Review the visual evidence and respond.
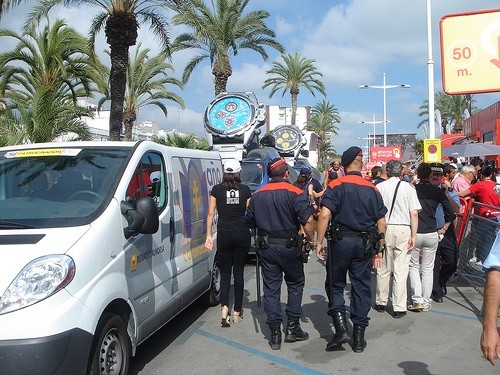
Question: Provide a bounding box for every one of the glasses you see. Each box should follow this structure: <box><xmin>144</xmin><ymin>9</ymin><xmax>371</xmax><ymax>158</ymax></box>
<box><xmin>470</xmin><ymin>171</ymin><xmax>476</xmax><ymax>179</ymax></box>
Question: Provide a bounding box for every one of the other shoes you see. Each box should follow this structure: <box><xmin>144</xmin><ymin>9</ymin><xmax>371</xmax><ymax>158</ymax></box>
<box><xmin>432</xmin><ymin>286</ymin><xmax>447</xmax><ymax>302</ymax></box>
<box><xmin>375</xmin><ymin>304</ymin><xmax>385</xmax><ymax>312</ymax></box>
<box><xmin>394</xmin><ymin>311</ymin><xmax>406</xmax><ymax>318</ymax></box>
<box><xmin>408</xmin><ymin>303</ymin><xmax>431</xmax><ymax>312</ymax></box>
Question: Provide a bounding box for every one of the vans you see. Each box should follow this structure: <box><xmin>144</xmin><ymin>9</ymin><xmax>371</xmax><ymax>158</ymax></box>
<box><xmin>0</xmin><ymin>139</ymin><xmax>226</xmax><ymax>375</ymax></box>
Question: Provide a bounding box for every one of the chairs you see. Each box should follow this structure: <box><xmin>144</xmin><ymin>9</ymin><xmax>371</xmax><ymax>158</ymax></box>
<box><xmin>22</xmin><ymin>172</ymin><xmax>51</xmax><ymax>204</ymax></box>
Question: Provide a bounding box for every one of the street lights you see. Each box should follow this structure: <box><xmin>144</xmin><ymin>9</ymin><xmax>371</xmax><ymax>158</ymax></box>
<box><xmin>357</xmin><ymin>72</ymin><xmax>412</xmax><ymax>148</ymax></box>
<box><xmin>357</xmin><ymin>132</ymin><xmax>382</xmax><ymax>163</ymax></box>
<box><xmin>357</xmin><ymin>113</ymin><xmax>391</xmax><ymax>147</ymax></box>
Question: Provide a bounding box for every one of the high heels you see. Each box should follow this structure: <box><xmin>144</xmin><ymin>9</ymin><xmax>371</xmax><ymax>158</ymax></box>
<box><xmin>233</xmin><ymin>310</ymin><xmax>243</xmax><ymax>323</ymax></box>
<box><xmin>221</xmin><ymin>304</ymin><xmax>231</xmax><ymax>327</ymax></box>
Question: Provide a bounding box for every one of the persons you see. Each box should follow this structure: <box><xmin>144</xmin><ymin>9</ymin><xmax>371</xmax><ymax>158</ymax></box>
<box><xmin>315</xmin><ymin>146</ymin><xmax>388</xmax><ymax>353</ymax></box>
<box><xmin>407</xmin><ymin>163</ymin><xmax>455</xmax><ymax>312</ymax></box>
<box><xmin>245</xmin><ymin>157</ymin><xmax>315</xmax><ymax>349</ymax></box>
<box><xmin>426</xmin><ymin>162</ymin><xmax>461</xmax><ymax>303</ymax></box>
<box><xmin>205</xmin><ymin>159</ymin><xmax>252</xmax><ymax>327</ymax></box>
<box><xmin>373</xmin><ymin>160</ymin><xmax>422</xmax><ymax>318</ymax></box>
<box><xmin>480</xmin><ymin>231</ymin><xmax>500</xmax><ymax>366</ymax></box>
<box><xmin>452</xmin><ymin>157</ymin><xmax>500</xmax><ymax>272</ymax></box>
<box><xmin>289</xmin><ymin>161</ymin><xmax>342</xmax><ymax>248</ymax></box>
<box><xmin>365</xmin><ymin>160</ymin><xmax>455</xmax><ymax>186</ymax></box>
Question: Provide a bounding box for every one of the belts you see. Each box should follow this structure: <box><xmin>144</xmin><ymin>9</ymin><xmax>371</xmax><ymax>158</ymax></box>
<box><xmin>342</xmin><ymin>230</ymin><xmax>362</xmax><ymax>238</ymax></box>
<box><xmin>267</xmin><ymin>237</ymin><xmax>288</xmax><ymax>245</ymax></box>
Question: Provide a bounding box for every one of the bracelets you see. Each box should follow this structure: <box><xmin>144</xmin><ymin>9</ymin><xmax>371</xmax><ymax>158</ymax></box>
<box><xmin>441</xmin><ymin>225</ymin><xmax>448</xmax><ymax>232</ymax></box>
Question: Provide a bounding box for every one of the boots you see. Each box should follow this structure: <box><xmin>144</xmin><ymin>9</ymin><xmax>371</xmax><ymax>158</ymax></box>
<box><xmin>353</xmin><ymin>324</ymin><xmax>367</xmax><ymax>353</ymax></box>
<box><xmin>326</xmin><ymin>311</ymin><xmax>351</xmax><ymax>349</ymax></box>
<box><xmin>285</xmin><ymin>316</ymin><xmax>309</xmax><ymax>340</ymax></box>
<box><xmin>269</xmin><ymin>322</ymin><xmax>282</xmax><ymax>350</ymax></box>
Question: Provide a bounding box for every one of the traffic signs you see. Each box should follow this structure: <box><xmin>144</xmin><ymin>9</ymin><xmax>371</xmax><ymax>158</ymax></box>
<box><xmin>439</xmin><ymin>7</ymin><xmax>500</xmax><ymax>96</ymax></box>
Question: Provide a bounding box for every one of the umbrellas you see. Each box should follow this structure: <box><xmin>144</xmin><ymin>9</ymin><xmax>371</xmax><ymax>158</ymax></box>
<box><xmin>442</xmin><ymin>139</ymin><xmax>500</xmax><ymax>159</ymax></box>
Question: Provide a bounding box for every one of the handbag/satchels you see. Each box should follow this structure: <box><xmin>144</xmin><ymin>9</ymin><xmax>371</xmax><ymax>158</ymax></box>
<box><xmin>365</xmin><ymin>232</ymin><xmax>385</xmax><ymax>256</ymax></box>
<box><xmin>297</xmin><ymin>237</ymin><xmax>310</xmax><ymax>263</ymax></box>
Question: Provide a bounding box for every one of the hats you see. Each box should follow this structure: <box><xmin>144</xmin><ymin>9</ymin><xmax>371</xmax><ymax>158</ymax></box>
<box><xmin>266</xmin><ymin>157</ymin><xmax>285</xmax><ymax>176</ymax></box>
<box><xmin>223</xmin><ymin>159</ymin><xmax>243</xmax><ymax>174</ymax></box>
<box><xmin>300</xmin><ymin>166</ymin><xmax>311</xmax><ymax>175</ymax></box>
<box><xmin>341</xmin><ymin>146</ymin><xmax>363</xmax><ymax>167</ymax></box>
<box><xmin>329</xmin><ymin>172</ymin><xmax>338</xmax><ymax>179</ymax></box>
<box><xmin>430</xmin><ymin>163</ymin><xmax>444</xmax><ymax>174</ymax></box>
